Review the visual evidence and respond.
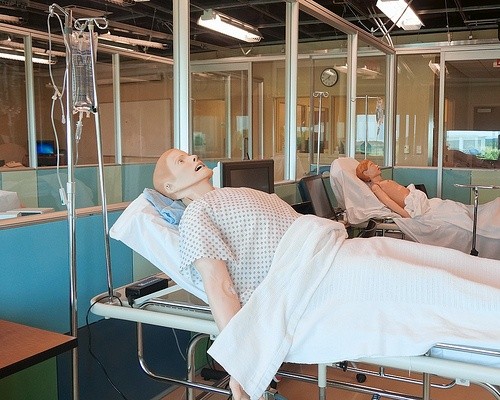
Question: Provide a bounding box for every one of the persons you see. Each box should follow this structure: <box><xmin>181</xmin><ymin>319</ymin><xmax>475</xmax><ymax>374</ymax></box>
<box><xmin>356</xmin><ymin>159</ymin><xmax>411</xmax><ymax>218</ymax></box>
<box><xmin>152</xmin><ymin>148</ymin><xmax>305</xmax><ymax>400</ymax></box>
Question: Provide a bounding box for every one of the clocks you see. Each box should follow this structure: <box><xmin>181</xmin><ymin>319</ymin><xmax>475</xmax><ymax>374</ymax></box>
<box><xmin>320</xmin><ymin>68</ymin><xmax>338</xmax><ymax>87</ymax></box>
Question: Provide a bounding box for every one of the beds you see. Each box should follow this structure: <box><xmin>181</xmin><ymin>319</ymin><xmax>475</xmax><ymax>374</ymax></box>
<box><xmin>88</xmin><ymin>188</ymin><xmax>500</xmax><ymax>400</ymax></box>
<box><xmin>329</xmin><ymin>156</ymin><xmax>500</xmax><ymax>261</ymax></box>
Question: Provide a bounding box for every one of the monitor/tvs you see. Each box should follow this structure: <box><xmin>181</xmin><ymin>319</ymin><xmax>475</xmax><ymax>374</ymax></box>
<box><xmin>36</xmin><ymin>140</ymin><xmax>55</xmax><ymax>155</ymax></box>
<box><xmin>213</xmin><ymin>159</ymin><xmax>275</xmax><ymax>196</ymax></box>
<box><xmin>299</xmin><ymin>174</ymin><xmax>338</xmax><ymax>223</ymax></box>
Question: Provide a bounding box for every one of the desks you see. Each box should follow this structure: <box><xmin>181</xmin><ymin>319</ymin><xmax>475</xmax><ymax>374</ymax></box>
<box><xmin>23</xmin><ymin>155</ymin><xmax>64</xmax><ymax>166</ymax></box>
<box><xmin>0</xmin><ymin>319</ymin><xmax>77</xmax><ymax>379</ymax></box>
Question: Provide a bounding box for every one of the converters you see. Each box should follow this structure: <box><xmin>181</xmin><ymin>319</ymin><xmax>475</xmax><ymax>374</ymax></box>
<box><xmin>125</xmin><ymin>277</ymin><xmax>168</xmax><ymax>299</ymax></box>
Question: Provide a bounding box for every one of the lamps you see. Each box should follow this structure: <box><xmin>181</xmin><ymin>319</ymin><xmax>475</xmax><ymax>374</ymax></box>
<box><xmin>374</xmin><ymin>0</ymin><xmax>425</xmax><ymax>31</ymax></box>
<box><xmin>195</xmin><ymin>8</ymin><xmax>264</xmax><ymax>44</ymax></box>
